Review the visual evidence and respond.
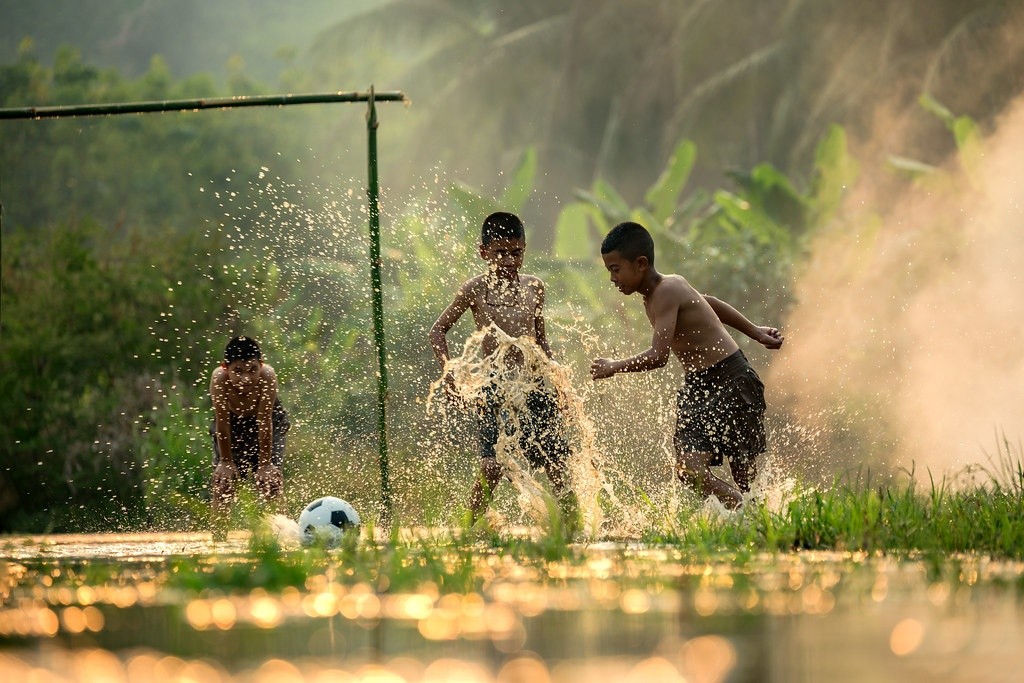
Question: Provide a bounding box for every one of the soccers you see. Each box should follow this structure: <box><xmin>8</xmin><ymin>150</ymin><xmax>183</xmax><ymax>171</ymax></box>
<box><xmin>298</xmin><ymin>497</ymin><xmax>361</xmax><ymax>550</ymax></box>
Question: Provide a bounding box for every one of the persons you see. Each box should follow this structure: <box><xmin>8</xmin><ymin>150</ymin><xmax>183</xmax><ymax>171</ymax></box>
<box><xmin>429</xmin><ymin>212</ymin><xmax>583</xmax><ymax>524</ymax></box>
<box><xmin>210</xmin><ymin>336</ymin><xmax>291</xmax><ymax>541</ymax></box>
<box><xmin>590</xmin><ymin>221</ymin><xmax>784</xmax><ymax>511</ymax></box>
